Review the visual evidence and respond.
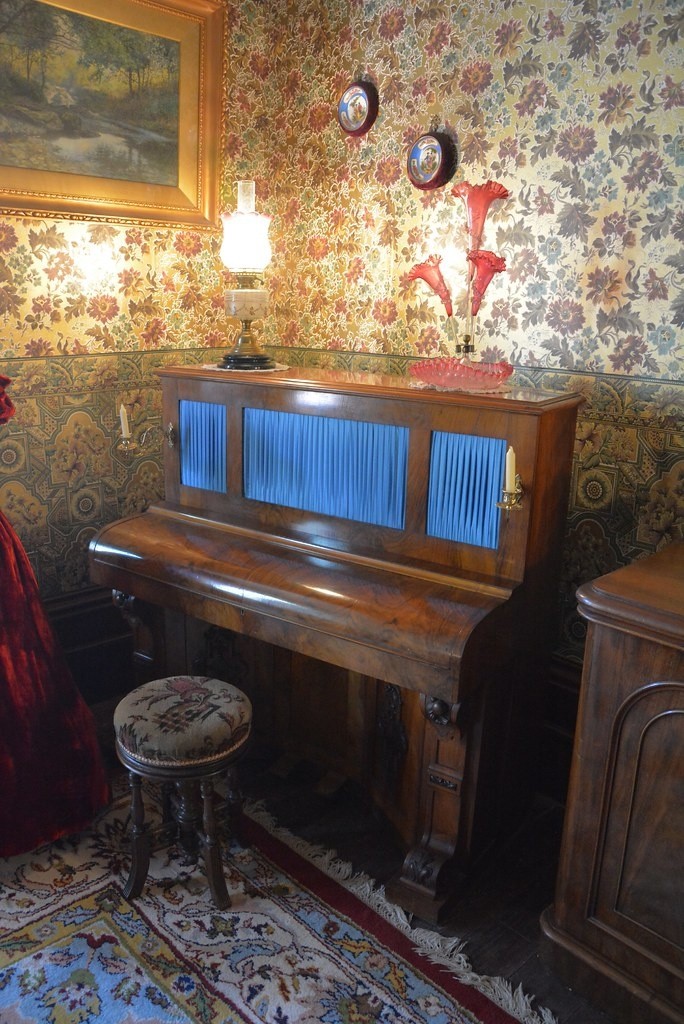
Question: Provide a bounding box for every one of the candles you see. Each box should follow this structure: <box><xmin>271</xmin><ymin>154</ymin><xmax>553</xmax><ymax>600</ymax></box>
<box><xmin>505</xmin><ymin>446</ymin><xmax>516</xmax><ymax>493</ymax></box>
<box><xmin>120</xmin><ymin>403</ymin><xmax>130</xmax><ymax>437</ymax></box>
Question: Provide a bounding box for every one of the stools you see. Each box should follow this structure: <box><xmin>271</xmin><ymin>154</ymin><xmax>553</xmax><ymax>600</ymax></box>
<box><xmin>112</xmin><ymin>676</ymin><xmax>254</xmax><ymax>911</ymax></box>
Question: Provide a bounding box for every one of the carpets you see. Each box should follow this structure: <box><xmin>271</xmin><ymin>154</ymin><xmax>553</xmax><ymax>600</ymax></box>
<box><xmin>0</xmin><ymin>763</ymin><xmax>563</xmax><ymax>1024</ymax></box>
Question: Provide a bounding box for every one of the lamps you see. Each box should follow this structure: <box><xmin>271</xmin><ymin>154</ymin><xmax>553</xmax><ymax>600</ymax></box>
<box><xmin>221</xmin><ymin>180</ymin><xmax>277</xmax><ymax>370</ymax></box>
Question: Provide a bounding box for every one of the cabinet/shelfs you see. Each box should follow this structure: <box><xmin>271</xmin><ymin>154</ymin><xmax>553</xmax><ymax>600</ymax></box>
<box><xmin>540</xmin><ymin>543</ymin><xmax>684</xmax><ymax>1024</ymax></box>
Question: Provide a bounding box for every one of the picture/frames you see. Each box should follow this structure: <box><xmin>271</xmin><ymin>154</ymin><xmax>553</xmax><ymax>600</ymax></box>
<box><xmin>2</xmin><ymin>1</ymin><xmax>231</xmax><ymax>229</ymax></box>
